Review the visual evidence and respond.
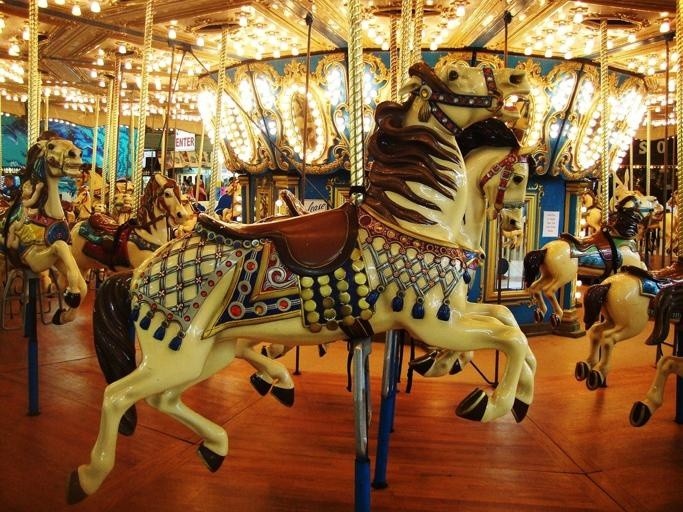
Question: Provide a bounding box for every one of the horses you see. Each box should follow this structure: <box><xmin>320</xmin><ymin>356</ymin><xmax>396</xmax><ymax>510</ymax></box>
<box><xmin>522</xmin><ymin>193</ymin><xmax>659</xmax><ymax>327</ymax></box>
<box><xmin>231</xmin><ymin>119</ymin><xmax>531</xmax><ymax>408</ymax></box>
<box><xmin>629</xmin><ymin>278</ymin><xmax>683</xmax><ymax>427</ymax></box>
<box><xmin>574</xmin><ymin>256</ymin><xmax>683</xmax><ymax>395</ymax></box>
<box><xmin>173</xmin><ymin>193</ymin><xmax>205</xmax><ymax>237</ymax></box>
<box><xmin>0</xmin><ymin>130</ymin><xmax>87</xmax><ymax>326</ymax></box>
<box><xmin>87</xmin><ymin>171</ymin><xmax>134</xmax><ymax>206</ymax></box>
<box><xmin>55</xmin><ymin>169</ymin><xmax>189</xmax><ymax>290</ymax></box>
<box><xmin>72</xmin><ymin>187</ymin><xmax>93</xmax><ymax>219</ymax></box>
<box><xmin>61</xmin><ymin>51</ymin><xmax>535</xmax><ymax>509</ymax></box>
<box><xmin>222</xmin><ymin>176</ymin><xmax>243</xmax><ymax>223</ymax></box>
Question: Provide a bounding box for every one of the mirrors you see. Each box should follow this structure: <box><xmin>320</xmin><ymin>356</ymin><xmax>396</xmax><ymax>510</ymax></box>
<box><xmin>326</xmin><ymin>177</ymin><xmax>350</xmax><ymax>209</ymax></box>
<box><xmin>255</xmin><ymin>188</ymin><xmax>273</xmax><ymax>221</ymax></box>
<box><xmin>477</xmin><ymin>183</ymin><xmax>545</xmax><ymax>308</ymax></box>
